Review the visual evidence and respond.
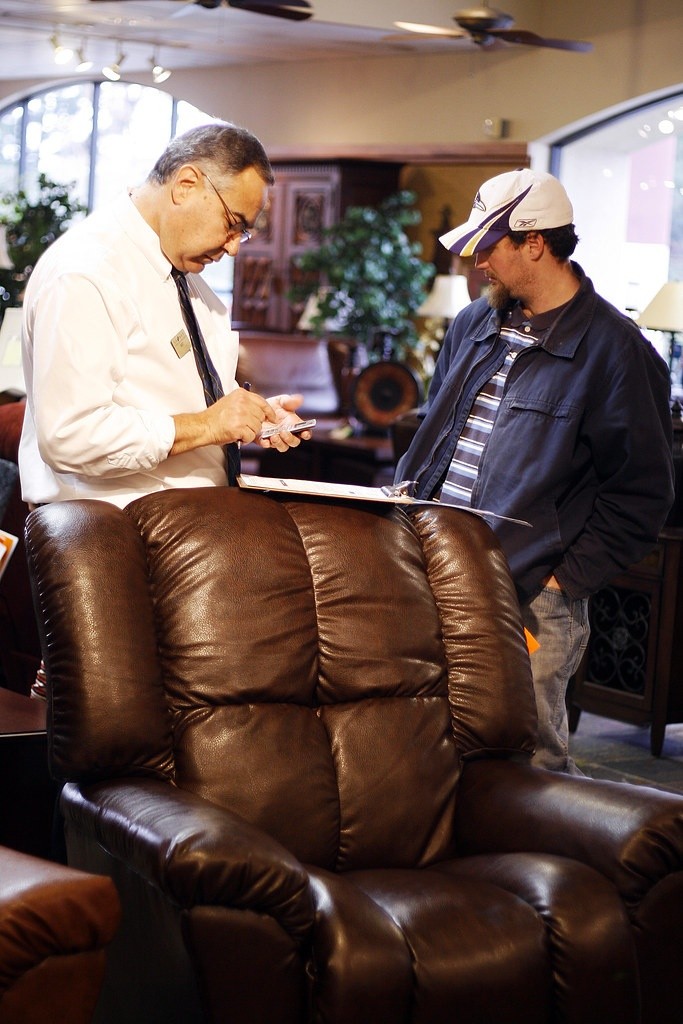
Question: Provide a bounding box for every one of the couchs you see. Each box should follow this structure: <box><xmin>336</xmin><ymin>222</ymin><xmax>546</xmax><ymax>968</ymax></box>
<box><xmin>21</xmin><ymin>488</ymin><xmax>683</xmax><ymax>1024</ymax></box>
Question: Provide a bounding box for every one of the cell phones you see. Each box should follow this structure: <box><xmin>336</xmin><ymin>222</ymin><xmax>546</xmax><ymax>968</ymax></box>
<box><xmin>262</xmin><ymin>418</ymin><xmax>317</xmax><ymax>439</ymax></box>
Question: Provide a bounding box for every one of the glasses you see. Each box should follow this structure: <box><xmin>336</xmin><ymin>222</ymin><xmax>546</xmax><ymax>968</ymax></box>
<box><xmin>199</xmin><ymin>171</ymin><xmax>253</xmax><ymax>246</ymax></box>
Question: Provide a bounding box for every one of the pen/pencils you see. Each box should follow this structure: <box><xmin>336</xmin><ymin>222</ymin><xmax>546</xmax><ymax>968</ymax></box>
<box><xmin>238</xmin><ymin>381</ymin><xmax>250</xmax><ymax>450</ymax></box>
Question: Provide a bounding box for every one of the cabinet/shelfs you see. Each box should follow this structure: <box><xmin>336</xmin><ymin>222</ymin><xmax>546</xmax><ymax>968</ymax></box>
<box><xmin>232</xmin><ymin>153</ymin><xmax>407</xmax><ymax>331</ymax></box>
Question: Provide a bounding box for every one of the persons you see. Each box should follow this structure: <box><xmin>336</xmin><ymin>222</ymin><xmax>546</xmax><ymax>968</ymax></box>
<box><xmin>17</xmin><ymin>114</ymin><xmax>311</xmax><ymax>511</ymax></box>
<box><xmin>394</xmin><ymin>166</ymin><xmax>679</xmax><ymax>769</ymax></box>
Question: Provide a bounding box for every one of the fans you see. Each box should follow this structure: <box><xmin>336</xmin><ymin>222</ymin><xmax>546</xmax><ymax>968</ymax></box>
<box><xmin>93</xmin><ymin>0</ymin><xmax>312</xmax><ymax>23</ymax></box>
<box><xmin>391</xmin><ymin>0</ymin><xmax>591</xmax><ymax>54</ymax></box>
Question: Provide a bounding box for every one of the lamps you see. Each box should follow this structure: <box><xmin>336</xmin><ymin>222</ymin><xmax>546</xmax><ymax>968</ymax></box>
<box><xmin>148</xmin><ymin>45</ymin><xmax>172</xmax><ymax>83</ymax></box>
<box><xmin>48</xmin><ymin>32</ymin><xmax>74</xmax><ymax>68</ymax></box>
<box><xmin>70</xmin><ymin>36</ymin><xmax>93</xmax><ymax>71</ymax></box>
<box><xmin>102</xmin><ymin>41</ymin><xmax>128</xmax><ymax>82</ymax></box>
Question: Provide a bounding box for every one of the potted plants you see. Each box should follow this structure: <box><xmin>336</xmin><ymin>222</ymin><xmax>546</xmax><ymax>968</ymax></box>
<box><xmin>290</xmin><ymin>191</ymin><xmax>436</xmax><ymax>405</ymax></box>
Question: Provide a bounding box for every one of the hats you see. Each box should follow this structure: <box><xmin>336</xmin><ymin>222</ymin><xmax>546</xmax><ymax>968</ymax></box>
<box><xmin>438</xmin><ymin>166</ymin><xmax>574</xmax><ymax>258</ymax></box>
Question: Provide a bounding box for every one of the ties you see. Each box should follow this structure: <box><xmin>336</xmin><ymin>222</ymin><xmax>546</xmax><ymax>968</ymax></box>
<box><xmin>168</xmin><ymin>267</ymin><xmax>242</xmax><ymax>486</ymax></box>
